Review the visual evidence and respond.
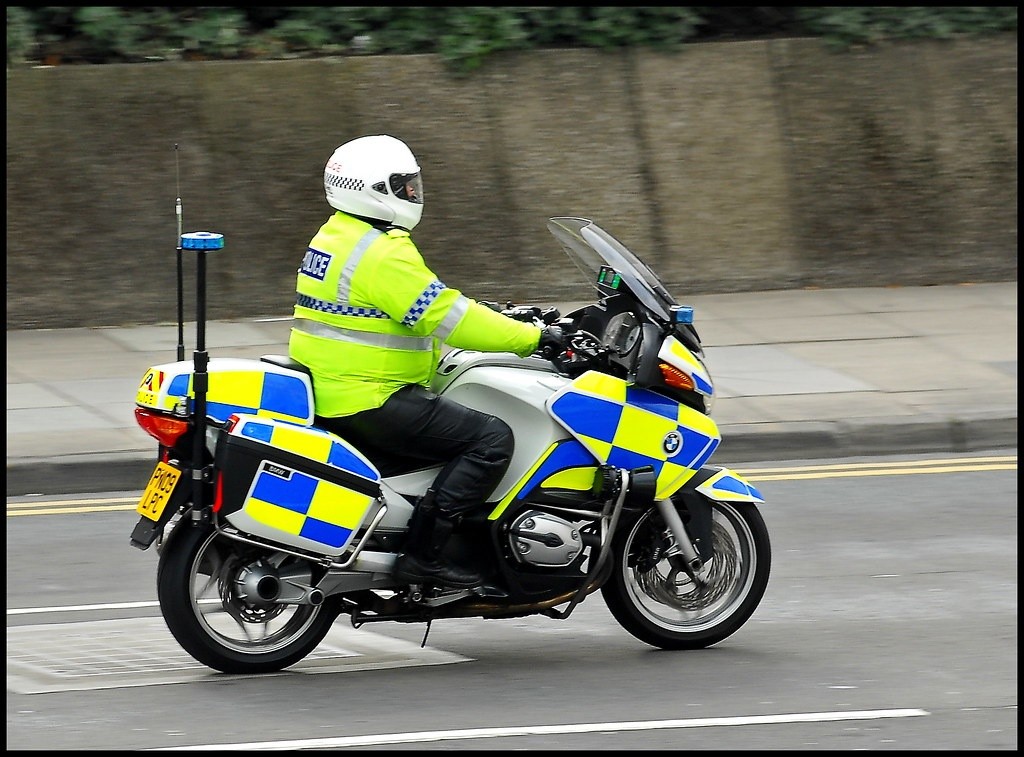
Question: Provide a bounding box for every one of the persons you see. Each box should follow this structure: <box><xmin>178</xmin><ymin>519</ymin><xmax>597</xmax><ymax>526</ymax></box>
<box><xmin>292</xmin><ymin>135</ymin><xmax>570</xmax><ymax>591</ymax></box>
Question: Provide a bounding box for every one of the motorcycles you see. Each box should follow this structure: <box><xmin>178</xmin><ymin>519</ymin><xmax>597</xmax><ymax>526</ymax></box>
<box><xmin>126</xmin><ymin>142</ymin><xmax>774</xmax><ymax>673</ymax></box>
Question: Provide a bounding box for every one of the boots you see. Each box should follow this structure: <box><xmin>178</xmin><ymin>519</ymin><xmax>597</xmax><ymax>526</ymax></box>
<box><xmin>391</xmin><ymin>495</ymin><xmax>484</xmax><ymax>590</ymax></box>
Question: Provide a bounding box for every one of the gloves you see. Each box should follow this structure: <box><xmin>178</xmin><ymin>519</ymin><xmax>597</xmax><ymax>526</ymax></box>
<box><xmin>539</xmin><ymin>323</ymin><xmax>567</xmax><ymax>361</ymax></box>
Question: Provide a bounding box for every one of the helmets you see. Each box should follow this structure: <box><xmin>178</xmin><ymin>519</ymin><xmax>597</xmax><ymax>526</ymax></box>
<box><xmin>323</xmin><ymin>134</ymin><xmax>424</xmax><ymax>230</ymax></box>
